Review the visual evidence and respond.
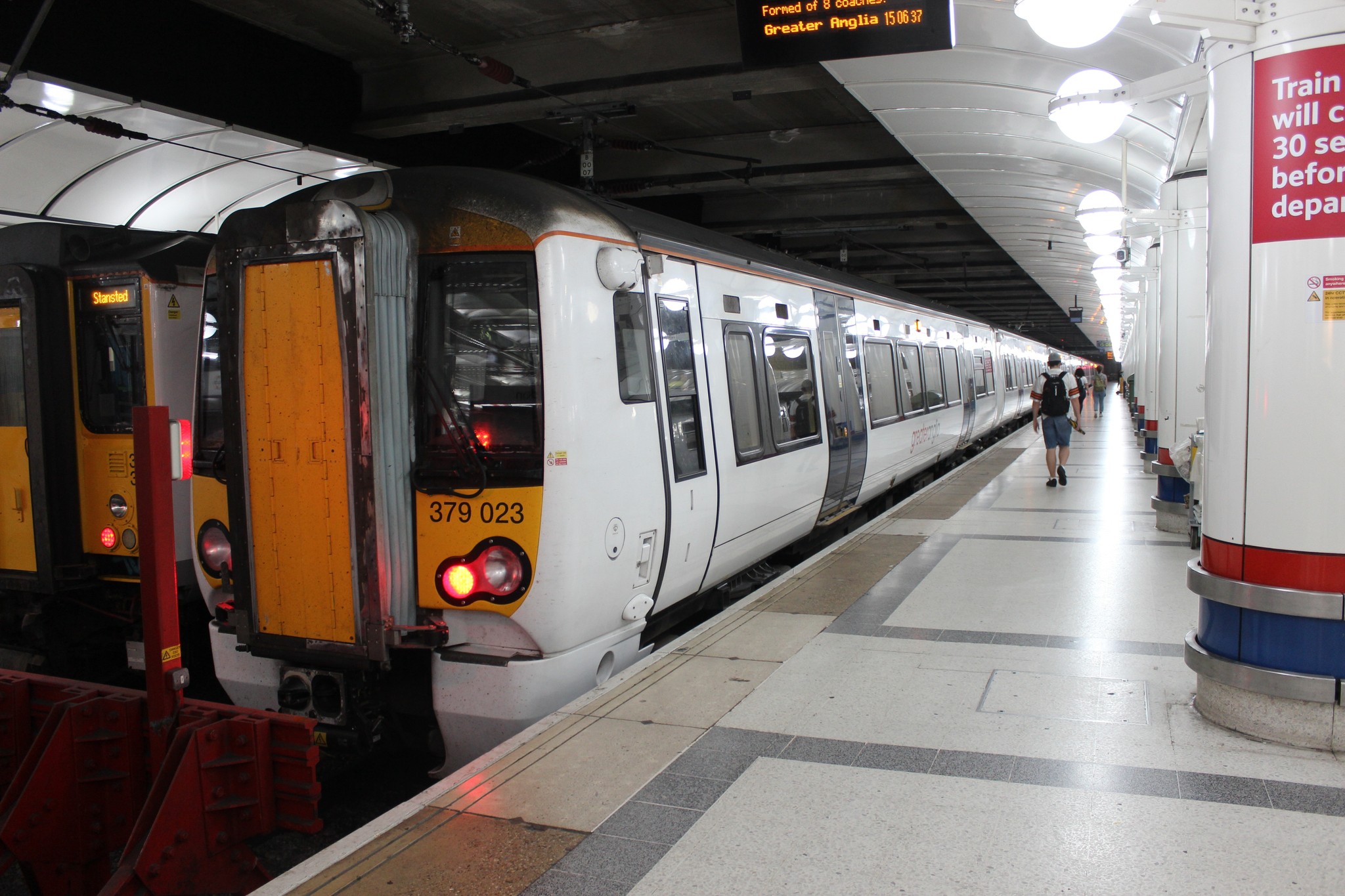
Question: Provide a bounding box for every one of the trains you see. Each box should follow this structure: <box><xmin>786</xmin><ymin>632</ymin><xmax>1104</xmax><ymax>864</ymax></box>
<box><xmin>185</xmin><ymin>161</ymin><xmax>1103</xmax><ymax>780</ymax></box>
<box><xmin>0</xmin><ymin>221</ymin><xmax>231</xmax><ymax>674</ymax></box>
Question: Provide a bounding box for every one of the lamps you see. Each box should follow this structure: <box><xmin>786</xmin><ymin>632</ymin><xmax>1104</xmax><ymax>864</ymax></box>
<box><xmin>1013</xmin><ymin>0</ymin><xmax>1196</xmax><ymax>66</ymax></box>
<box><xmin>1075</xmin><ymin>190</ymin><xmax>1180</xmax><ymax>363</ymax></box>
<box><xmin>1049</xmin><ymin>60</ymin><xmax>1209</xmax><ymax>144</ymax></box>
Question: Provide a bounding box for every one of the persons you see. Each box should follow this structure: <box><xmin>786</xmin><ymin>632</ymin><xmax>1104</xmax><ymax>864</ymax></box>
<box><xmin>1029</xmin><ymin>352</ymin><xmax>1081</xmax><ymax>488</ymax></box>
<box><xmin>1117</xmin><ymin>370</ymin><xmax>1124</xmax><ymax>388</ymax></box>
<box><xmin>1090</xmin><ymin>365</ymin><xmax>1108</xmax><ymax>418</ymax></box>
<box><xmin>1074</xmin><ymin>368</ymin><xmax>1090</xmax><ymax>415</ymax></box>
<box><xmin>788</xmin><ymin>379</ymin><xmax>817</xmax><ymax>441</ymax></box>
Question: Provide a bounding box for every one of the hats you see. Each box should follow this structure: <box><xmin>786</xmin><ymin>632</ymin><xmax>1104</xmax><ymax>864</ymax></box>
<box><xmin>1048</xmin><ymin>352</ymin><xmax>1062</xmax><ymax>366</ymax></box>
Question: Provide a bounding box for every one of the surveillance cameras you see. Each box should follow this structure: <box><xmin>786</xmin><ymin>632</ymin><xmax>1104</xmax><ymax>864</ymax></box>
<box><xmin>1116</xmin><ymin>247</ymin><xmax>1131</xmax><ymax>261</ymax></box>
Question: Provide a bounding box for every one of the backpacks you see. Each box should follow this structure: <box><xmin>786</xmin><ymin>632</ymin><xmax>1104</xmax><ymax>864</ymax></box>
<box><xmin>1040</xmin><ymin>371</ymin><xmax>1070</xmax><ymax>416</ymax></box>
<box><xmin>1075</xmin><ymin>376</ymin><xmax>1084</xmax><ymax>392</ymax></box>
<box><xmin>1094</xmin><ymin>374</ymin><xmax>1105</xmax><ymax>392</ymax></box>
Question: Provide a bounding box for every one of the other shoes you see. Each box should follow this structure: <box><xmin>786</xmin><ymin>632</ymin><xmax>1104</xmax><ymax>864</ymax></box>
<box><xmin>1094</xmin><ymin>411</ymin><xmax>1097</xmax><ymax>418</ymax></box>
<box><xmin>1057</xmin><ymin>465</ymin><xmax>1067</xmax><ymax>486</ymax></box>
<box><xmin>1099</xmin><ymin>413</ymin><xmax>1103</xmax><ymax>417</ymax></box>
<box><xmin>1046</xmin><ymin>478</ymin><xmax>1057</xmax><ymax>487</ymax></box>
